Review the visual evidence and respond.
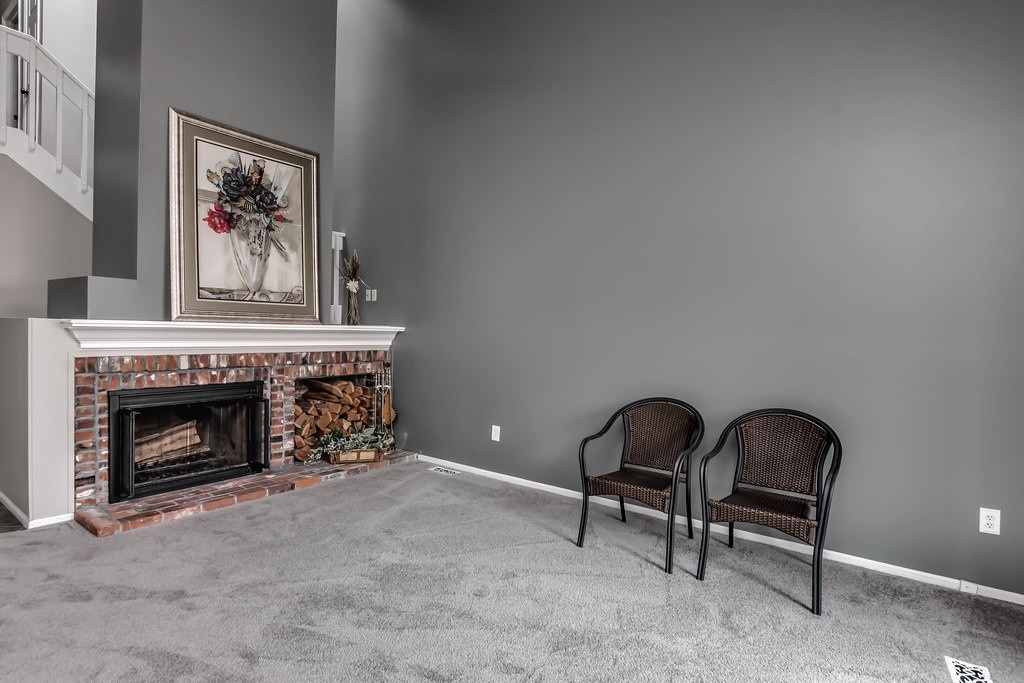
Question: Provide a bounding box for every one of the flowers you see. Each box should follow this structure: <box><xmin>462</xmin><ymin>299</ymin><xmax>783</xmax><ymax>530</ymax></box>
<box><xmin>202</xmin><ymin>151</ymin><xmax>294</xmax><ymax>263</ymax></box>
<box><xmin>338</xmin><ymin>248</ymin><xmax>370</xmax><ymax>294</ymax></box>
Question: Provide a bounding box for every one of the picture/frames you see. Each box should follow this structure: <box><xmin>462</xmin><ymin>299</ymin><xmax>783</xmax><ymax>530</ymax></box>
<box><xmin>168</xmin><ymin>106</ymin><xmax>322</xmax><ymax>326</ymax></box>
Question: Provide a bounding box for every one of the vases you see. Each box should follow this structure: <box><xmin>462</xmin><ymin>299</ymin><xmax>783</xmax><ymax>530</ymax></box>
<box><xmin>347</xmin><ymin>290</ymin><xmax>360</xmax><ymax>324</ymax></box>
<box><xmin>230</xmin><ymin>216</ymin><xmax>276</xmax><ymax>294</ymax></box>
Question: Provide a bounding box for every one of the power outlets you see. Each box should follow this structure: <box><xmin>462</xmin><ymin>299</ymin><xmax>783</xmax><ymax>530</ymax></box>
<box><xmin>491</xmin><ymin>425</ymin><xmax>500</xmax><ymax>441</ymax></box>
<box><xmin>978</xmin><ymin>508</ymin><xmax>1003</xmax><ymax>536</ymax></box>
<box><xmin>365</xmin><ymin>290</ymin><xmax>372</xmax><ymax>302</ymax></box>
<box><xmin>372</xmin><ymin>289</ymin><xmax>377</xmax><ymax>301</ymax></box>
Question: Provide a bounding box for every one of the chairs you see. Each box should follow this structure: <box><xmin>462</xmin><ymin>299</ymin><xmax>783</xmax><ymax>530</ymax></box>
<box><xmin>576</xmin><ymin>396</ymin><xmax>706</xmax><ymax>575</ymax></box>
<box><xmin>696</xmin><ymin>407</ymin><xmax>843</xmax><ymax>616</ymax></box>
